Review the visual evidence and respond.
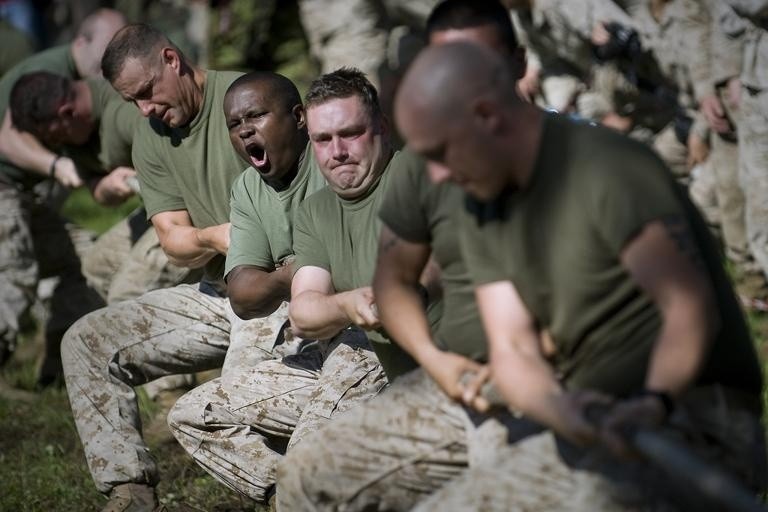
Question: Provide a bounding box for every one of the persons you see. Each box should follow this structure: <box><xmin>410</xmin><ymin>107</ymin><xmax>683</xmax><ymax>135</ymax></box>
<box><xmin>275</xmin><ymin>2</ymin><xmax>527</xmax><ymax>512</ymax></box>
<box><xmin>0</xmin><ymin>2</ymin><xmax>425</xmax><ymax>511</ymax></box>
<box><xmin>394</xmin><ymin>40</ymin><xmax>767</xmax><ymax>510</ymax></box>
<box><xmin>511</xmin><ymin>1</ymin><xmax>767</xmax><ymax>309</ymax></box>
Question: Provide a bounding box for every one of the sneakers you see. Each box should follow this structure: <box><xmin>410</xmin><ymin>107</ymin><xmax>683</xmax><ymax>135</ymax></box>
<box><xmin>102</xmin><ymin>483</ymin><xmax>168</xmax><ymax>512</ymax></box>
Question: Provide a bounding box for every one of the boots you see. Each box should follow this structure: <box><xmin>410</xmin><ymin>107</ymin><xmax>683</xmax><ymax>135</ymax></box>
<box><xmin>142</xmin><ymin>387</ymin><xmax>191</xmax><ymax>447</ymax></box>
